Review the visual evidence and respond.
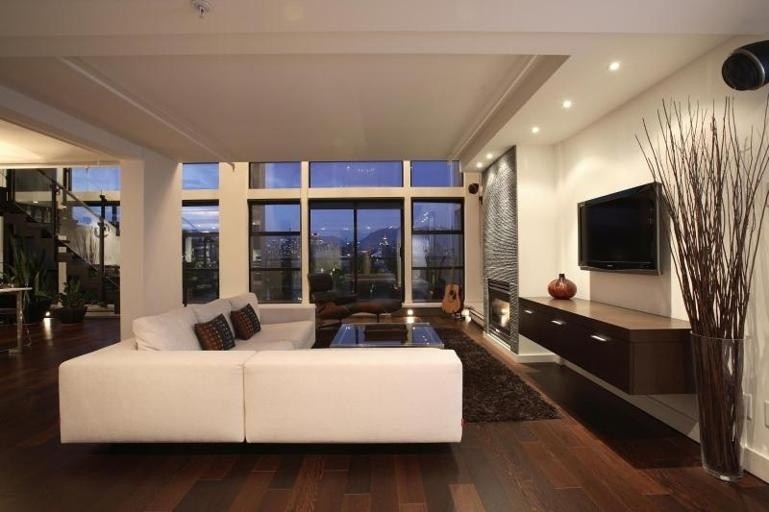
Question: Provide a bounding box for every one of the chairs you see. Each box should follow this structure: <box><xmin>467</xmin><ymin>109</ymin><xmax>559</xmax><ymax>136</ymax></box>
<box><xmin>308</xmin><ymin>273</ymin><xmax>402</xmax><ymax>323</ymax></box>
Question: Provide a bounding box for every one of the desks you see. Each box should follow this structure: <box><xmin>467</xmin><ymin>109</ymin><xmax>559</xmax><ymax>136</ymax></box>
<box><xmin>0</xmin><ymin>286</ymin><xmax>33</xmax><ymax>324</ymax></box>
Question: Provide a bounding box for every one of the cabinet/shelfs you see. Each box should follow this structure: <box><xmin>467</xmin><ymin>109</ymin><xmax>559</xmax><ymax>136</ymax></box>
<box><xmin>518</xmin><ymin>296</ymin><xmax>691</xmax><ymax>395</ymax></box>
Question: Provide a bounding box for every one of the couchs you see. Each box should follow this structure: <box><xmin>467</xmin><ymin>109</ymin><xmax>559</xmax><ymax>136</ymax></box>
<box><xmin>58</xmin><ymin>303</ymin><xmax>463</xmax><ymax>444</ymax></box>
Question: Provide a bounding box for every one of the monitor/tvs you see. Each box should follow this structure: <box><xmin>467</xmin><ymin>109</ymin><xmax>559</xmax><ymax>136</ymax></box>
<box><xmin>577</xmin><ymin>182</ymin><xmax>664</xmax><ymax>277</ymax></box>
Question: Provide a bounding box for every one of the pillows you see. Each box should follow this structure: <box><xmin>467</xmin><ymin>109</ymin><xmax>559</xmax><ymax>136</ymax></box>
<box><xmin>229</xmin><ymin>293</ymin><xmax>260</xmax><ymax>322</ymax></box>
<box><xmin>230</xmin><ymin>304</ymin><xmax>260</xmax><ymax>340</ymax></box>
<box><xmin>189</xmin><ymin>298</ymin><xmax>235</xmax><ymax>338</ymax></box>
<box><xmin>194</xmin><ymin>313</ymin><xmax>235</xmax><ymax>350</ymax></box>
<box><xmin>132</xmin><ymin>307</ymin><xmax>201</xmax><ymax>351</ymax></box>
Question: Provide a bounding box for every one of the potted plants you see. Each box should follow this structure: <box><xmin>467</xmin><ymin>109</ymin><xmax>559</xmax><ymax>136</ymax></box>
<box><xmin>630</xmin><ymin>95</ymin><xmax>769</xmax><ymax>481</ymax></box>
<box><xmin>60</xmin><ymin>275</ymin><xmax>88</xmax><ymax>324</ymax></box>
<box><xmin>6</xmin><ymin>248</ymin><xmax>53</xmax><ymax>323</ymax></box>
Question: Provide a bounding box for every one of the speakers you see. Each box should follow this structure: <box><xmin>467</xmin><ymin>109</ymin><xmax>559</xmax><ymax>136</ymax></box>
<box><xmin>468</xmin><ymin>183</ymin><xmax>479</xmax><ymax>194</ymax></box>
<box><xmin>722</xmin><ymin>40</ymin><xmax>769</xmax><ymax>91</ymax></box>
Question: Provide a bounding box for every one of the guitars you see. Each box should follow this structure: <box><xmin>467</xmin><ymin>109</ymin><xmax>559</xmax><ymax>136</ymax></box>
<box><xmin>442</xmin><ymin>258</ymin><xmax>460</xmax><ymax>313</ymax></box>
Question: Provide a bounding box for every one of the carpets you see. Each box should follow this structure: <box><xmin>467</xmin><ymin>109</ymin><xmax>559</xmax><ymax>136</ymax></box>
<box><xmin>311</xmin><ymin>328</ymin><xmax>562</xmax><ymax>422</ymax></box>
